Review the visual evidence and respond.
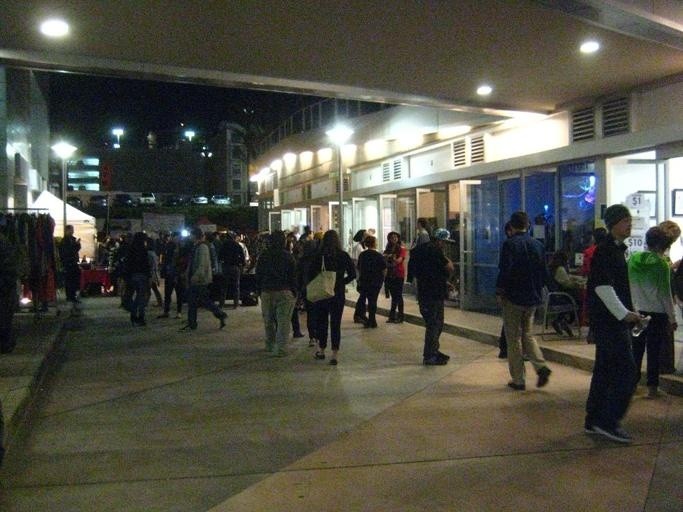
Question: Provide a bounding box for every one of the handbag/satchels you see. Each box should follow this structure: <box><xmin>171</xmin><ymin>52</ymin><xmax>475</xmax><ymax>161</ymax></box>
<box><xmin>304</xmin><ymin>273</ymin><xmax>336</xmax><ymax>301</ymax></box>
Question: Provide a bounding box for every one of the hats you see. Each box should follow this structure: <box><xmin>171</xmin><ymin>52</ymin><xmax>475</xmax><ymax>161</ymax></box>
<box><xmin>432</xmin><ymin>227</ymin><xmax>455</xmax><ymax>243</ymax></box>
<box><xmin>604</xmin><ymin>206</ymin><xmax>628</xmax><ymax>228</ymax></box>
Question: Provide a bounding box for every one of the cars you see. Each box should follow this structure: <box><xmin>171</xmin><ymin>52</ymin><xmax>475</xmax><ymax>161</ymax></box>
<box><xmin>162</xmin><ymin>195</ymin><xmax>231</xmax><ymax>207</ymax></box>
<box><xmin>108</xmin><ymin>194</ymin><xmax>133</xmax><ymax>208</ymax></box>
<box><xmin>88</xmin><ymin>195</ymin><xmax>108</xmax><ymax>208</ymax></box>
<box><xmin>67</xmin><ymin>197</ymin><xmax>82</xmax><ymax>209</ymax></box>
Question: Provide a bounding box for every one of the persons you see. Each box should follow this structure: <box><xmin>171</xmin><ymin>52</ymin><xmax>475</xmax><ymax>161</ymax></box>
<box><xmin>60</xmin><ymin>225</ymin><xmax>82</xmax><ymax>304</ymax></box>
<box><xmin>411</xmin><ymin>228</ymin><xmax>455</xmax><ymax>365</ymax></box>
<box><xmin>351</xmin><ymin>229</ymin><xmax>407</xmax><ymax>328</ymax></box>
<box><xmin>585</xmin><ymin>204</ymin><xmax>644</xmax><ymax>445</ymax></box>
<box><xmin>254</xmin><ymin>229</ymin><xmax>357</xmax><ymax>366</ymax></box>
<box><xmin>499</xmin><ymin>211</ymin><xmax>552</xmax><ymax>390</ymax></box>
<box><xmin>583</xmin><ymin>226</ymin><xmax>608</xmax><ymax>344</ymax></box>
<box><xmin>545</xmin><ymin>247</ymin><xmax>582</xmax><ymax>336</ymax></box>
<box><xmin>415</xmin><ymin>217</ymin><xmax>430</xmax><ymax>247</ymax></box>
<box><xmin>625</xmin><ymin>220</ymin><xmax>681</xmax><ymax>398</ymax></box>
<box><xmin>100</xmin><ymin>227</ymin><xmax>259</xmax><ymax>332</ymax></box>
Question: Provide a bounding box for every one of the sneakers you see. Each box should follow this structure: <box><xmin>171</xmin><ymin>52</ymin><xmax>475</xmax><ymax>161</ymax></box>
<box><xmin>537</xmin><ymin>366</ymin><xmax>550</xmax><ymax>388</ymax></box>
<box><xmin>510</xmin><ymin>381</ymin><xmax>527</xmax><ymax>391</ymax></box>
<box><xmin>423</xmin><ymin>353</ymin><xmax>451</xmax><ymax>366</ymax></box>
<box><xmin>316</xmin><ymin>351</ymin><xmax>325</xmax><ymax>360</ymax></box>
<box><xmin>584</xmin><ymin>424</ymin><xmax>635</xmax><ymax>445</ymax></box>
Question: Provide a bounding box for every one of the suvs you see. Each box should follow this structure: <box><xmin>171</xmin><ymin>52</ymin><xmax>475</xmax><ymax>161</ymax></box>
<box><xmin>136</xmin><ymin>192</ymin><xmax>156</xmax><ymax>207</ymax></box>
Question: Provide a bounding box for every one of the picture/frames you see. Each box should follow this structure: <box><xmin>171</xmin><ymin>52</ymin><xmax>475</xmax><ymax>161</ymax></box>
<box><xmin>672</xmin><ymin>189</ymin><xmax>683</xmax><ymax>218</ymax></box>
<box><xmin>637</xmin><ymin>190</ymin><xmax>658</xmax><ymax>218</ymax></box>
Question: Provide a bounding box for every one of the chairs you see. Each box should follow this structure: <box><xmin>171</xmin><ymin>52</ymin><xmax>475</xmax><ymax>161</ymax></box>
<box><xmin>538</xmin><ymin>285</ymin><xmax>582</xmax><ymax>339</ymax></box>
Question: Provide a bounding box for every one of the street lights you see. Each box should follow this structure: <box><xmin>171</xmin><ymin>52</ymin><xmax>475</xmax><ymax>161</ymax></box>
<box><xmin>326</xmin><ymin>121</ymin><xmax>351</xmax><ymax>248</ymax></box>
<box><xmin>243</xmin><ymin>106</ymin><xmax>254</xmax><ymax>204</ymax></box>
<box><xmin>51</xmin><ymin>140</ymin><xmax>80</xmax><ymax>236</ymax></box>
<box><xmin>199</xmin><ymin>145</ymin><xmax>212</xmax><ymax>202</ymax></box>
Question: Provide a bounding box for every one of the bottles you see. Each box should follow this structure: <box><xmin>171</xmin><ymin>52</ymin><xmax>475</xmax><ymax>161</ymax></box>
<box><xmin>81</xmin><ymin>255</ymin><xmax>87</xmax><ymax>263</ymax></box>
<box><xmin>631</xmin><ymin>315</ymin><xmax>652</xmax><ymax>339</ymax></box>
